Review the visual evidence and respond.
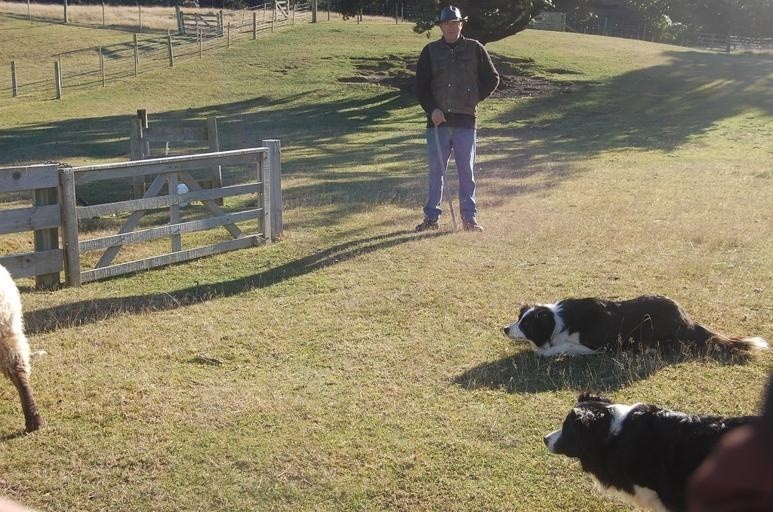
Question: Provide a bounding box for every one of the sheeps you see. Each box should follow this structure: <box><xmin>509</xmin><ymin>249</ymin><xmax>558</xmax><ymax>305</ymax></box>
<box><xmin>1</xmin><ymin>262</ymin><xmax>43</xmax><ymax>436</ymax></box>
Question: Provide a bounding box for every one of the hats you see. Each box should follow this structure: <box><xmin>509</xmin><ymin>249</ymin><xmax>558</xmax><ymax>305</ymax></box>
<box><xmin>435</xmin><ymin>5</ymin><xmax>468</xmax><ymax>24</ymax></box>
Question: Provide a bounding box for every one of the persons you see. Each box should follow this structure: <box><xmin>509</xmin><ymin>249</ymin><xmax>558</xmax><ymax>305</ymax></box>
<box><xmin>412</xmin><ymin>6</ymin><xmax>501</xmax><ymax>234</ymax></box>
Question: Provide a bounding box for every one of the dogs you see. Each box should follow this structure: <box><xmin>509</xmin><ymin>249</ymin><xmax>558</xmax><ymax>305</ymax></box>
<box><xmin>543</xmin><ymin>391</ymin><xmax>757</xmax><ymax>512</ymax></box>
<box><xmin>502</xmin><ymin>290</ymin><xmax>769</xmax><ymax>362</ymax></box>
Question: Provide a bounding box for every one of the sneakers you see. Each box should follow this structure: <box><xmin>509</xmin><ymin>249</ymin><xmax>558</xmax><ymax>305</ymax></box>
<box><xmin>463</xmin><ymin>221</ymin><xmax>483</xmax><ymax>233</ymax></box>
<box><xmin>416</xmin><ymin>217</ymin><xmax>439</xmax><ymax>231</ymax></box>
